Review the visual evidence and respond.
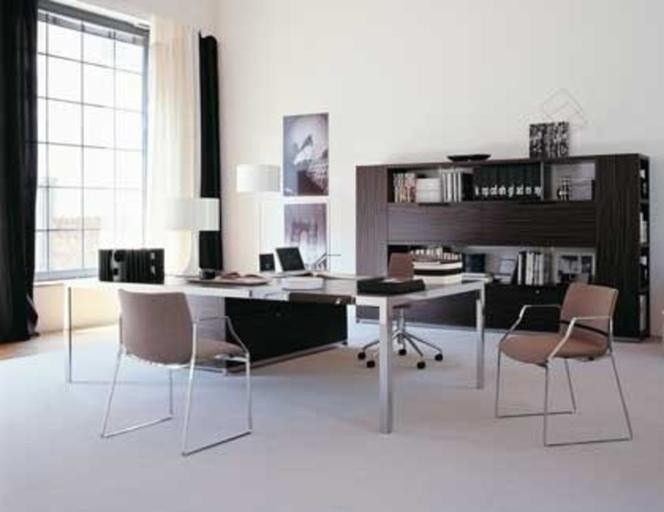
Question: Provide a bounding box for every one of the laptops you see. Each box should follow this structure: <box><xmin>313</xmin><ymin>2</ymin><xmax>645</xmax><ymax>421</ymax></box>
<box><xmin>257</xmin><ymin>252</ymin><xmax>276</xmax><ymax>273</ymax></box>
<box><xmin>276</xmin><ymin>245</ymin><xmax>312</xmax><ymax>276</ymax></box>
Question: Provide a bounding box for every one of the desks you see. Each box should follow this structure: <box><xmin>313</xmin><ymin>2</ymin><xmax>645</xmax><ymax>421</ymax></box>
<box><xmin>62</xmin><ymin>276</ymin><xmax>485</xmax><ymax>435</ymax></box>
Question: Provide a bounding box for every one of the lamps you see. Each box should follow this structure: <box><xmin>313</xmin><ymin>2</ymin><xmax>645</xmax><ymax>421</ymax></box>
<box><xmin>172</xmin><ymin>198</ymin><xmax>220</xmax><ymax>277</ymax></box>
<box><xmin>236</xmin><ymin>163</ymin><xmax>281</xmax><ymax>273</ymax></box>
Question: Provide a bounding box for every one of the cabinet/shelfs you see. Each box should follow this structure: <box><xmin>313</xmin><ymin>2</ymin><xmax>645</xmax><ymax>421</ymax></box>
<box><xmin>354</xmin><ymin>152</ymin><xmax>653</xmax><ymax>344</ymax></box>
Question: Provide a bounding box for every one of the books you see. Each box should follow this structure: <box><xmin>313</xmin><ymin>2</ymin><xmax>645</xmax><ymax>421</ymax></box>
<box><xmin>441</xmin><ymin>164</ymin><xmax>542</xmax><ymax>203</ymax></box>
<box><xmin>513</xmin><ymin>250</ymin><xmax>550</xmax><ymax>286</ymax></box>
<box><xmin>462</xmin><ymin>272</ymin><xmax>493</xmax><ymax>285</ymax></box>
<box><xmin>274</xmin><ymin>269</ymin><xmax>357</xmax><ymax>279</ymax></box>
<box><xmin>557</xmin><ymin>176</ymin><xmax>594</xmax><ymax>201</ymax></box>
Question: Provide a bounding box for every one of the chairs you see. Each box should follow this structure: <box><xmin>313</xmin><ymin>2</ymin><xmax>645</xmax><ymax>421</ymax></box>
<box><xmin>357</xmin><ymin>252</ymin><xmax>444</xmax><ymax>370</ymax></box>
<box><xmin>494</xmin><ymin>282</ymin><xmax>634</xmax><ymax>447</ymax></box>
<box><xmin>99</xmin><ymin>288</ymin><xmax>254</xmax><ymax>458</ymax></box>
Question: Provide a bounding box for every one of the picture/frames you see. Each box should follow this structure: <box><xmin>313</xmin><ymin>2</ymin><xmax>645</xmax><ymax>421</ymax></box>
<box><xmin>282</xmin><ymin>112</ymin><xmax>330</xmax><ymax>197</ymax></box>
<box><xmin>282</xmin><ymin>203</ymin><xmax>329</xmax><ymax>274</ymax></box>
